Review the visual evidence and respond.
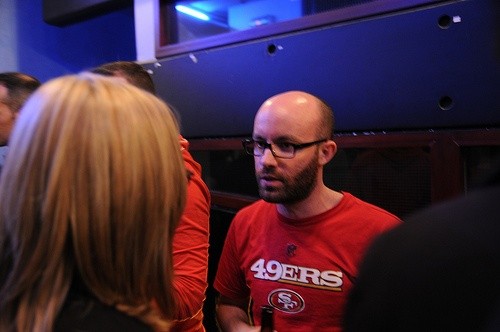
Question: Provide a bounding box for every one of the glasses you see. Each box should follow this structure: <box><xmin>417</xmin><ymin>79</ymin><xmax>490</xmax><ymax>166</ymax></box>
<box><xmin>242</xmin><ymin>138</ymin><xmax>328</xmax><ymax>159</ymax></box>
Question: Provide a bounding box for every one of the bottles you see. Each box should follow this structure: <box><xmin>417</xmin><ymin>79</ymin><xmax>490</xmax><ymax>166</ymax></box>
<box><xmin>259</xmin><ymin>306</ymin><xmax>275</xmax><ymax>332</ymax></box>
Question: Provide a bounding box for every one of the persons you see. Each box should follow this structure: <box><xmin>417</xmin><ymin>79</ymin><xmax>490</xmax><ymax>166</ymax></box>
<box><xmin>213</xmin><ymin>91</ymin><xmax>406</xmax><ymax>332</ymax></box>
<box><xmin>341</xmin><ymin>190</ymin><xmax>500</xmax><ymax>332</ymax></box>
<box><xmin>0</xmin><ymin>69</ymin><xmax>187</xmax><ymax>332</ymax></box>
<box><xmin>0</xmin><ymin>72</ymin><xmax>42</xmax><ymax>147</ymax></box>
<box><xmin>91</xmin><ymin>61</ymin><xmax>211</xmax><ymax>332</ymax></box>
